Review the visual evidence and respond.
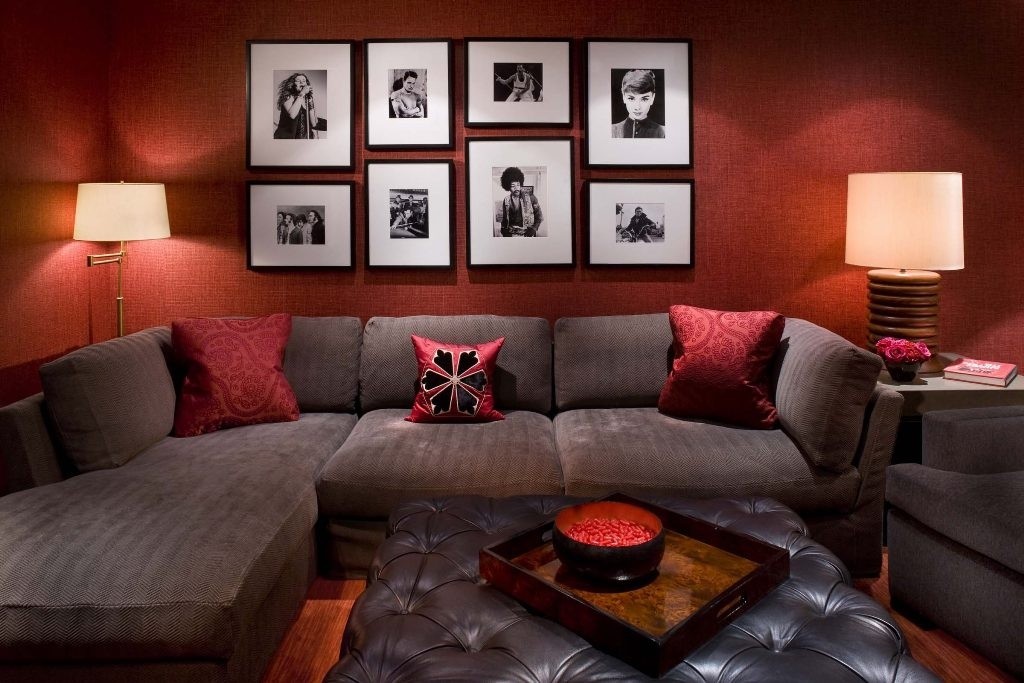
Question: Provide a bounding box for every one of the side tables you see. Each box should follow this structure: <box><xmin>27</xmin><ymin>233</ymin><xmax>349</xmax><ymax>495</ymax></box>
<box><xmin>878</xmin><ymin>352</ymin><xmax>1024</xmax><ymax>465</ymax></box>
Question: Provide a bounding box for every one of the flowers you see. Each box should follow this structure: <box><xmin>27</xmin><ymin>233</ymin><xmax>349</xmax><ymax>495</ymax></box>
<box><xmin>875</xmin><ymin>337</ymin><xmax>931</xmax><ymax>363</ymax></box>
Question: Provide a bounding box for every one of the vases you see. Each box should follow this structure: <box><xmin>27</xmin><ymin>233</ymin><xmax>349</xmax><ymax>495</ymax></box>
<box><xmin>884</xmin><ymin>360</ymin><xmax>923</xmax><ymax>384</ymax></box>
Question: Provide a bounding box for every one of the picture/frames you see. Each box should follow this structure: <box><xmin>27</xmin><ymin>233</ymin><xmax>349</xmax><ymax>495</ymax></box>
<box><xmin>361</xmin><ymin>36</ymin><xmax>453</xmax><ymax>149</ymax></box>
<box><xmin>463</xmin><ymin>36</ymin><xmax>573</xmax><ymax>127</ymax></box>
<box><xmin>245</xmin><ymin>179</ymin><xmax>356</xmax><ymax>273</ymax></box>
<box><xmin>585</xmin><ymin>178</ymin><xmax>694</xmax><ymax>268</ymax></box>
<box><xmin>245</xmin><ymin>39</ymin><xmax>354</xmax><ymax>173</ymax></box>
<box><xmin>364</xmin><ymin>158</ymin><xmax>455</xmax><ymax>270</ymax></box>
<box><xmin>581</xmin><ymin>36</ymin><xmax>694</xmax><ymax>170</ymax></box>
<box><xmin>465</xmin><ymin>136</ymin><xmax>577</xmax><ymax>267</ymax></box>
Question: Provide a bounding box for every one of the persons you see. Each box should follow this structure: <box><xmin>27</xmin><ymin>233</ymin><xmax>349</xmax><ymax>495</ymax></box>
<box><xmin>391</xmin><ymin>71</ymin><xmax>424</xmax><ymax>118</ymax></box>
<box><xmin>612</xmin><ymin>69</ymin><xmax>665</xmax><ymax>139</ymax></box>
<box><xmin>620</xmin><ymin>206</ymin><xmax>656</xmax><ymax>245</ymax></box>
<box><xmin>495</xmin><ymin>63</ymin><xmax>543</xmax><ymax>102</ymax></box>
<box><xmin>276</xmin><ymin>210</ymin><xmax>326</xmax><ymax>245</ymax></box>
<box><xmin>273</xmin><ymin>73</ymin><xmax>319</xmax><ymax>140</ymax></box>
<box><xmin>390</xmin><ymin>194</ymin><xmax>429</xmax><ymax>231</ymax></box>
<box><xmin>500</xmin><ymin>167</ymin><xmax>543</xmax><ymax>237</ymax></box>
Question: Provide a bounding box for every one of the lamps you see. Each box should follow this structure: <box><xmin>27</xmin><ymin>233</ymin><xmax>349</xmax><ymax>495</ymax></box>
<box><xmin>845</xmin><ymin>172</ymin><xmax>964</xmax><ymax>373</ymax></box>
<box><xmin>72</xmin><ymin>180</ymin><xmax>171</xmax><ymax>337</ymax></box>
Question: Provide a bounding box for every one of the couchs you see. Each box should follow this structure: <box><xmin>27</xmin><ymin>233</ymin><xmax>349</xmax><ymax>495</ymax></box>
<box><xmin>885</xmin><ymin>404</ymin><xmax>1023</xmax><ymax>683</ymax></box>
<box><xmin>315</xmin><ymin>313</ymin><xmax>903</xmax><ymax>582</ymax></box>
<box><xmin>0</xmin><ymin>312</ymin><xmax>362</xmax><ymax>683</ymax></box>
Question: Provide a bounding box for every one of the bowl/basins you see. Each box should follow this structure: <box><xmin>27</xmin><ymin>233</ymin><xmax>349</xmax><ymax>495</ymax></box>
<box><xmin>552</xmin><ymin>501</ymin><xmax>665</xmax><ymax>581</ymax></box>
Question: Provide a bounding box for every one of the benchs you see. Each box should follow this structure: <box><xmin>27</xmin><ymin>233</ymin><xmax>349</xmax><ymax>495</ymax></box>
<box><xmin>324</xmin><ymin>493</ymin><xmax>943</xmax><ymax>683</ymax></box>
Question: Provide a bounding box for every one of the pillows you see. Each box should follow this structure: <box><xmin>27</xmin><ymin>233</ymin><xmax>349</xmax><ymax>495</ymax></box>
<box><xmin>170</xmin><ymin>313</ymin><xmax>301</xmax><ymax>438</ymax></box>
<box><xmin>404</xmin><ymin>331</ymin><xmax>508</xmax><ymax>421</ymax></box>
<box><xmin>657</xmin><ymin>306</ymin><xmax>787</xmax><ymax>429</ymax></box>
